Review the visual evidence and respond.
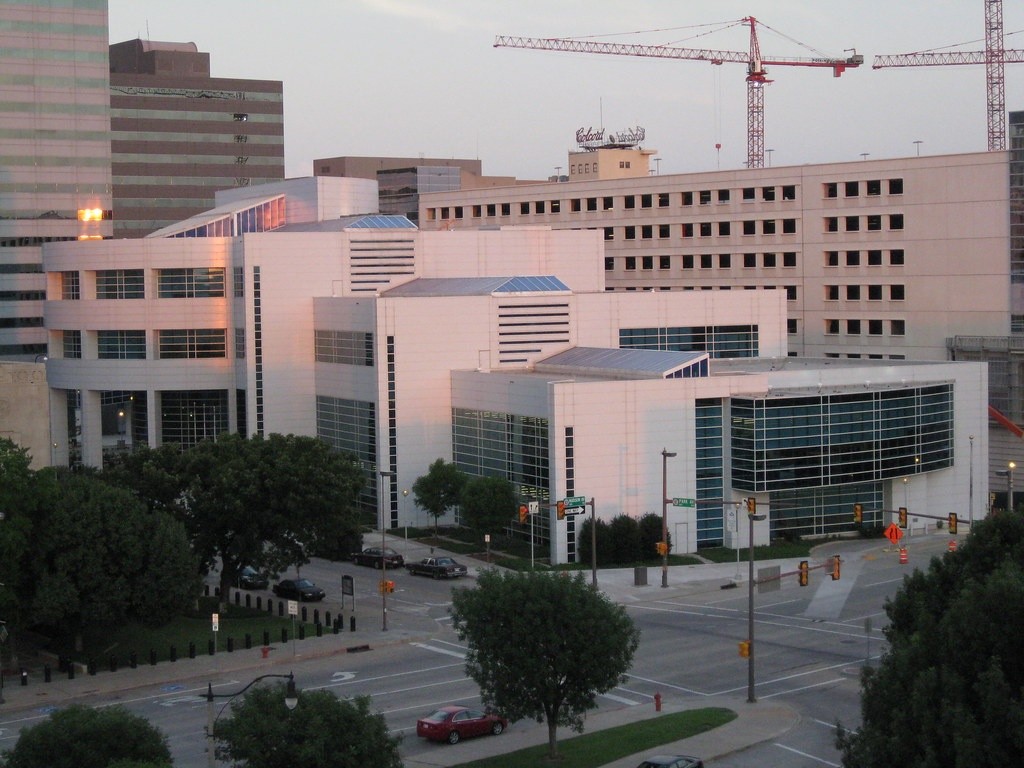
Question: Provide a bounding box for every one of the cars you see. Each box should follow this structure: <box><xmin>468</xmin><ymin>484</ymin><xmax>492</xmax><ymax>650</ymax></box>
<box><xmin>350</xmin><ymin>545</ymin><xmax>405</xmax><ymax>570</ymax></box>
<box><xmin>272</xmin><ymin>577</ymin><xmax>326</xmax><ymax>602</ymax></box>
<box><xmin>405</xmin><ymin>555</ymin><xmax>468</xmax><ymax>580</ymax></box>
<box><xmin>220</xmin><ymin>565</ymin><xmax>270</xmax><ymax>590</ymax></box>
<box><xmin>415</xmin><ymin>704</ymin><xmax>508</xmax><ymax>745</ymax></box>
<box><xmin>635</xmin><ymin>755</ymin><xmax>705</xmax><ymax>768</ymax></box>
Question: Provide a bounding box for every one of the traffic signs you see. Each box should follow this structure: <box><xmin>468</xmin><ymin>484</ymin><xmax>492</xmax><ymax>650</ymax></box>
<box><xmin>564</xmin><ymin>505</ymin><xmax>585</xmax><ymax>516</ymax></box>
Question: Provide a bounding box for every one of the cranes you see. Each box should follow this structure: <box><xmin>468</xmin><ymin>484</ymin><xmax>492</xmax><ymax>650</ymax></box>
<box><xmin>869</xmin><ymin>0</ymin><xmax>1024</xmax><ymax>151</ymax></box>
<box><xmin>492</xmin><ymin>14</ymin><xmax>865</xmax><ymax>171</ymax></box>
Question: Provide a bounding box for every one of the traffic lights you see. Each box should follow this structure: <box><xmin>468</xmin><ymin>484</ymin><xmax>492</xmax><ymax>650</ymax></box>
<box><xmin>738</xmin><ymin>641</ymin><xmax>750</xmax><ymax>658</ymax></box>
<box><xmin>948</xmin><ymin>512</ymin><xmax>958</xmax><ymax>534</ymax></box>
<box><xmin>386</xmin><ymin>581</ymin><xmax>395</xmax><ymax>593</ymax></box>
<box><xmin>656</xmin><ymin>541</ymin><xmax>666</xmax><ymax>555</ymax></box>
<box><xmin>798</xmin><ymin>561</ymin><xmax>809</xmax><ymax>587</ymax></box>
<box><xmin>898</xmin><ymin>506</ymin><xmax>908</xmax><ymax>528</ymax></box>
<box><xmin>519</xmin><ymin>505</ymin><xmax>528</xmax><ymax>523</ymax></box>
<box><xmin>853</xmin><ymin>503</ymin><xmax>862</xmax><ymax>524</ymax></box>
<box><xmin>746</xmin><ymin>497</ymin><xmax>757</xmax><ymax>515</ymax></box>
<box><xmin>829</xmin><ymin>555</ymin><xmax>840</xmax><ymax>581</ymax></box>
<box><xmin>556</xmin><ymin>500</ymin><xmax>566</xmax><ymax>520</ymax></box>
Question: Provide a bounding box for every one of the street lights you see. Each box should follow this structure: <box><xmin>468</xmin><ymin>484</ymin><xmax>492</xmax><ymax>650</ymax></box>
<box><xmin>554</xmin><ymin>166</ymin><xmax>562</xmax><ymax>176</ymax></box>
<box><xmin>653</xmin><ymin>157</ymin><xmax>663</xmax><ymax>173</ymax></box>
<box><xmin>860</xmin><ymin>153</ymin><xmax>870</xmax><ymax>161</ymax></box>
<box><xmin>912</xmin><ymin>140</ymin><xmax>924</xmax><ymax>157</ymax></box>
<box><xmin>745</xmin><ymin>512</ymin><xmax>769</xmax><ymax>703</ymax></box>
<box><xmin>659</xmin><ymin>446</ymin><xmax>679</xmax><ymax>588</ymax></box>
<box><xmin>198</xmin><ymin>668</ymin><xmax>299</xmax><ymax>768</ymax></box>
<box><xmin>378</xmin><ymin>470</ymin><xmax>396</xmax><ymax>631</ymax></box>
<box><xmin>994</xmin><ymin>469</ymin><xmax>1014</xmax><ymax>513</ymax></box>
<box><xmin>764</xmin><ymin>149</ymin><xmax>774</xmax><ymax>167</ymax></box>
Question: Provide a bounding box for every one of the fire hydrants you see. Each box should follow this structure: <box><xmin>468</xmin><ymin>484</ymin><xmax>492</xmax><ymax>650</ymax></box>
<box><xmin>653</xmin><ymin>691</ymin><xmax>664</xmax><ymax>712</ymax></box>
